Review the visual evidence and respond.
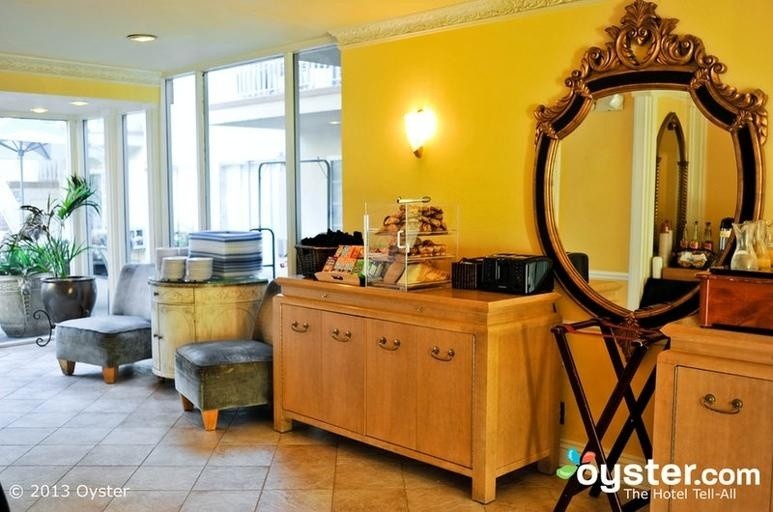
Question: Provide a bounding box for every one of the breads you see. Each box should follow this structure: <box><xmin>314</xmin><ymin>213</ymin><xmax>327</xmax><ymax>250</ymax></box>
<box><xmin>383</xmin><ymin>260</ymin><xmax>449</xmax><ymax>285</ymax></box>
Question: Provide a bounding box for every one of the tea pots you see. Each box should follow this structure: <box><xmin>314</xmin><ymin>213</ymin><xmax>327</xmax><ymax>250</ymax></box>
<box><xmin>729</xmin><ymin>218</ymin><xmax>771</xmax><ymax>274</ymax></box>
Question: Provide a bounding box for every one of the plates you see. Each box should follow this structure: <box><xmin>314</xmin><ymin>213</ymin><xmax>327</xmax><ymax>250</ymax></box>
<box><xmin>152</xmin><ymin>246</ymin><xmax>214</xmax><ymax>282</ymax></box>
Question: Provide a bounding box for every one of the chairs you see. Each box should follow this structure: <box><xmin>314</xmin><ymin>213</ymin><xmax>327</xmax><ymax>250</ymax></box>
<box><xmin>175</xmin><ymin>280</ymin><xmax>281</xmax><ymax>432</ymax></box>
<box><xmin>55</xmin><ymin>263</ymin><xmax>158</xmax><ymax>383</ymax></box>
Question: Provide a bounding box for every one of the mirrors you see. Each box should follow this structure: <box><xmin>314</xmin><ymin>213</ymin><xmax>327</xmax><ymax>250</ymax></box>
<box><xmin>654</xmin><ymin>111</ymin><xmax>689</xmax><ymax>266</ymax></box>
<box><xmin>531</xmin><ymin>0</ymin><xmax>766</xmax><ymax>331</ymax></box>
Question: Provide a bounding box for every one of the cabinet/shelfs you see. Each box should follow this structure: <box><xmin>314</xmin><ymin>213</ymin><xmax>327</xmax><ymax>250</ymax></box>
<box><xmin>652</xmin><ymin>326</ymin><xmax>773</xmax><ymax>512</ymax></box>
<box><xmin>363</xmin><ymin>202</ymin><xmax>459</xmax><ymax>291</ymax></box>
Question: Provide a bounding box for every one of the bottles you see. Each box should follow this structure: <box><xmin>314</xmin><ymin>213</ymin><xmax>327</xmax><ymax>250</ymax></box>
<box><xmin>649</xmin><ymin>219</ymin><xmax>714</xmax><ymax>280</ymax></box>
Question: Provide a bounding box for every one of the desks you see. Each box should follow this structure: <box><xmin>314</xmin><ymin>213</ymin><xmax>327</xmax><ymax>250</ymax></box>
<box><xmin>147</xmin><ymin>276</ymin><xmax>268</xmax><ymax>388</ymax></box>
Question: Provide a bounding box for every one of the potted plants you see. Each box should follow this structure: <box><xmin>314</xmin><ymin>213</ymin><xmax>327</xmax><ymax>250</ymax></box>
<box><xmin>0</xmin><ymin>174</ymin><xmax>96</xmax><ymax>346</ymax></box>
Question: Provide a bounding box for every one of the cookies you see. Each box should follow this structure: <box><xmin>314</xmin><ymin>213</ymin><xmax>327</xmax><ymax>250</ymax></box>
<box><xmin>388</xmin><ymin>237</ymin><xmax>447</xmax><ymax>256</ymax></box>
<box><xmin>384</xmin><ymin>205</ymin><xmax>447</xmax><ymax>232</ymax></box>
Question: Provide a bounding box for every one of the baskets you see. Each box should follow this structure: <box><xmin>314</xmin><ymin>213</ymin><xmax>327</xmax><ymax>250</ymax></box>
<box><xmin>294</xmin><ymin>242</ymin><xmax>338</xmax><ymax>278</ymax></box>
